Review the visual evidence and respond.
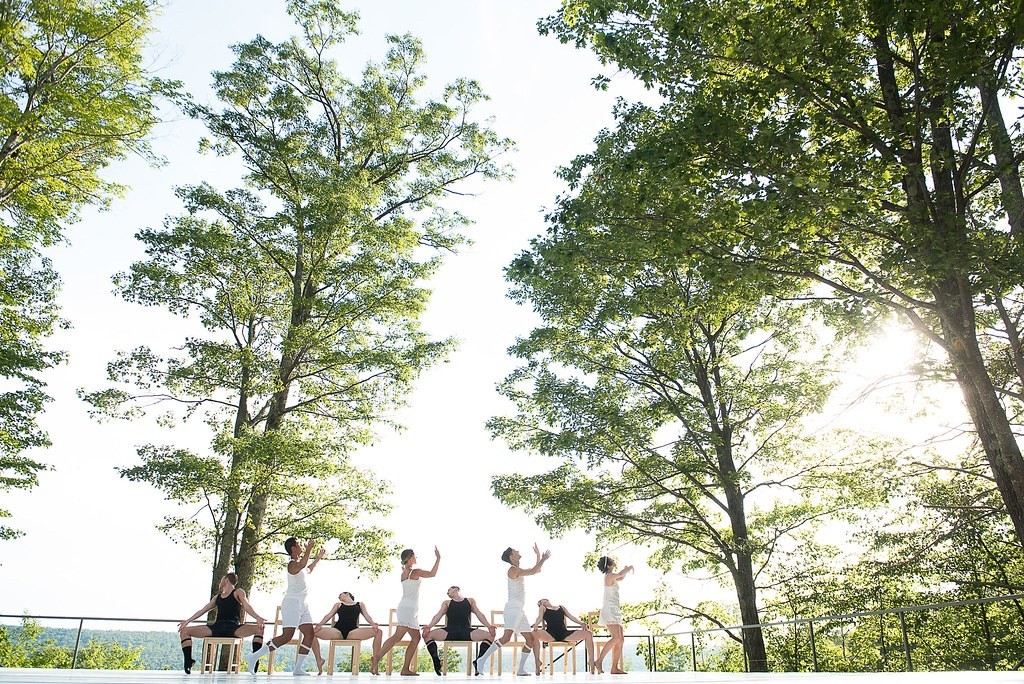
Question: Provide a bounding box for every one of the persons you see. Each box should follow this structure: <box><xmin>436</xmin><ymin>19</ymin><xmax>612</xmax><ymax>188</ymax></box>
<box><xmin>531</xmin><ymin>599</ymin><xmax>596</xmax><ymax>675</ymax></box>
<box><xmin>311</xmin><ymin>592</ymin><xmax>382</xmax><ymax>676</ymax></box>
<box><xmin>473</xmin><ymin>542</ymin><xmax>552</xmax><ymax>676</ymax></box>
<box><xmin>242</xmin><ymin>537</ymin><xmax>325</xmax><ymax>676</ymax></box>
<box><xmin>370</xmin><ymin>545</ymin><xmax>440</xmax><ymax>676</ymax></box>
<box><xmin>419</xmin><ymin>587</ymin><xmax>497</xmax><ymax>677</ymax></box>
<box><xmin>177</xmin><ymin>572</ymin><xmax>270</xmax><ymax>675</ymax></box>
<box><xmin>594</xmin><ymin>556</ymin><xmax>634</xmax><ymax>675</ymax></box>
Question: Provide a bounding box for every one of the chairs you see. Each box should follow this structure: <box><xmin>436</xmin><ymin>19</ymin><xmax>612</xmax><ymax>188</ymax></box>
<box><xmin>443</xmin><ymin>614</ymin><xmax>472</xmax><ymax>676</ymax></box>
<box><xmin>541</xmin><ymin>614</ymin><xmax>576</xmax><ymax>675</ymax></box>
<box><xmin>201</xmin><ymin>597</ymin><xmax>249</xmax><ymax>675</ymax></box>
<box><xmin>490</xmin><ymin>611</ymin><xmax>525</xmax><ymax>675</ymax></box>
<box><xmin>386</xmin><ymin>608</ymin><xmax>417</xmax><ymax>675</ymax></box>
<box><xmin>268</xmin><ymin>606</ymin><xmax>302</xmax><ymax>675</ymax></box>
<box><xmin>327</xmin><ymin>615</ymin><xmax>362</xmax><ymax>675</ymax></box>
<box><xmin>588</xmin><ymin>612</ymin><xmax>623</xmax><ymax>674</ymax></box>
